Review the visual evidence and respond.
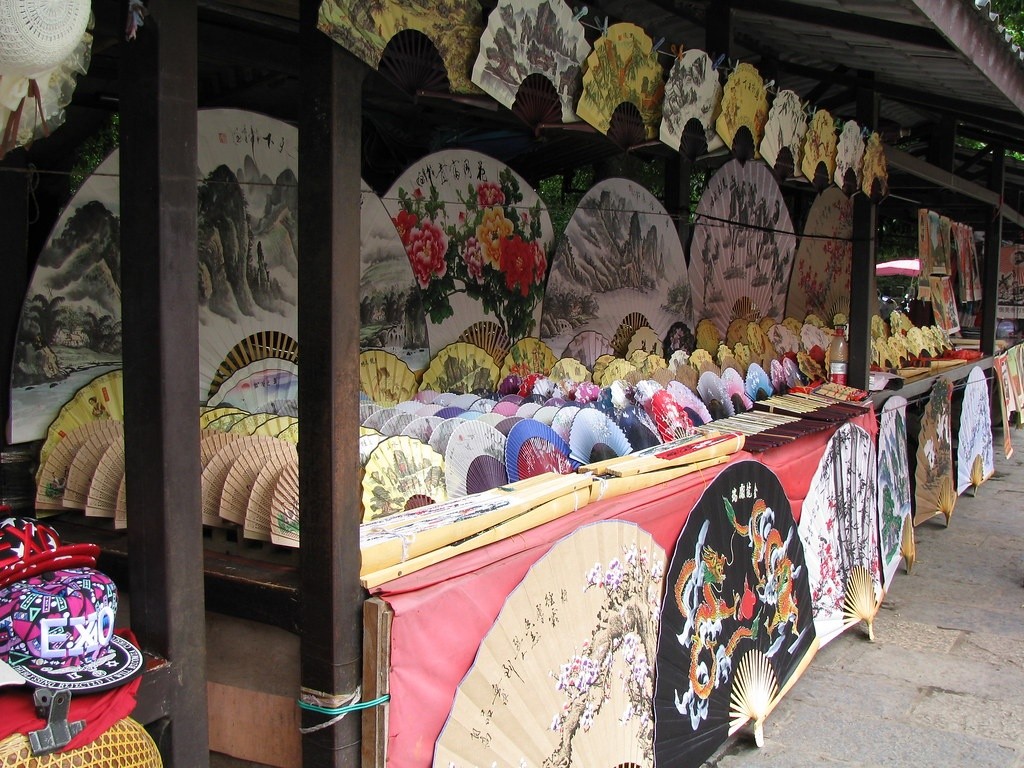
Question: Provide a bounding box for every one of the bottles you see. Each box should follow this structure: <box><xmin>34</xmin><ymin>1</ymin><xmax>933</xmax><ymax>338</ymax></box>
<box><xmin>829</xmin><ymin>324</ymin><xmax>849</xmax><ymax>385</ymax></box>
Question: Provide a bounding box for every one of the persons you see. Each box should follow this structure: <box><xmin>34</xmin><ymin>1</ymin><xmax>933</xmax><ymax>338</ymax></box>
<box><xmin>976</xmin><ymin>240</ymin><xmax>984</xmax><ymax>275</ymax></box>
<box><xmin>1014</xmin><ymin>251</ymin><xmax>1024</xmax><ymax>265</ymax></box>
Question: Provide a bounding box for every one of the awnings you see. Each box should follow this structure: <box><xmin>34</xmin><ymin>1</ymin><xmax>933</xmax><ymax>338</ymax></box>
<box><xmin>875</xmin><ymin>259</ymin><xmax>919</xmax><ymax>277</ymax></box>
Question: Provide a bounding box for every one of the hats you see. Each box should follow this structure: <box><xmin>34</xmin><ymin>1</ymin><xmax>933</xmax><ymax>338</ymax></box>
<box><xmin>2</xmin><ymin>568</ymin><xmax>145</xmax><ymax>694</ymax></box>
<box><xmin>0</xmin><ymin>505</ymin><xmax>102</xmax><ymax>591</ymax></box>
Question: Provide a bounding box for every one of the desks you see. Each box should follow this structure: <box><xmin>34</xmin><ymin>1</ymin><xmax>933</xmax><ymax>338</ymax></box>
<box><xmin>28</xmin><ymin>340</ymin><xmax>1024</xmax><ymax>768</ymax></box>
